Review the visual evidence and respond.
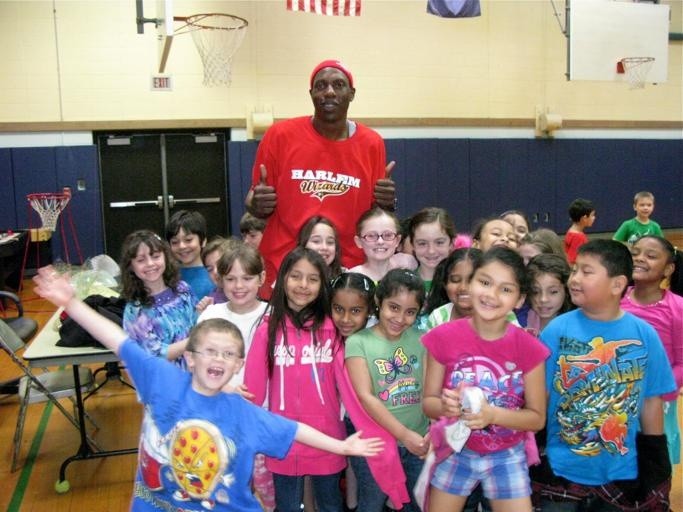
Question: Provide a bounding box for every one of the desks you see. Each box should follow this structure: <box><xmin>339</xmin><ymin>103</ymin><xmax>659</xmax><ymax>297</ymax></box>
<box><xmin>23</xmin><ymin>306</ymin><xmax>138</xmax><ymax>493</ymax></box>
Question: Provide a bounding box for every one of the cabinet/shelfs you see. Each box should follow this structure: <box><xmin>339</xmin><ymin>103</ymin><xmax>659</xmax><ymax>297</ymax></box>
<box><xmin>92</xmin><ymin>128</ymin><xmax>232</xmax><ymax>266</ymax></box>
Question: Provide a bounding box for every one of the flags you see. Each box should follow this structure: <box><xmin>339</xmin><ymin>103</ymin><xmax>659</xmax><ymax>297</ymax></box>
<box><xmin>285</xmin><ymin>0</ymin><xmax>363</xmax><ymax>19</ymax></box>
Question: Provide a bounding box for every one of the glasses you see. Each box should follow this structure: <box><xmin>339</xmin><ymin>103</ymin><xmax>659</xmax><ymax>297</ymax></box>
<box><xmin>191</xmin><ymin>346</ymin><xmax>241</xmax><ymax>368</ymax></box>
<box><xmin>359</xmin><ymin>230</ymin><xmax>398</xmax><ymax>243</ymax></box>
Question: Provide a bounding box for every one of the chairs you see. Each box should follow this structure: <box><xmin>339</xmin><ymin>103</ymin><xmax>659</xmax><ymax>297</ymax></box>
<box><xmin>1</xmin><ymin>319</ymin><xmax>107</xmax><ymax>473</ymax></box>
<box><xmin>1</xmin><ymin>290</ymin><xmax>38</xmax><ymax>395</ymax></box>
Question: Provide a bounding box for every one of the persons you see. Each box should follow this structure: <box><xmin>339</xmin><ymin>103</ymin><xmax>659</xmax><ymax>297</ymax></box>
<box><xmin>121</xmin><ymin>191</ymin><xmax>664</xmax><ymax>372</ymax></box>
<box><xmin>539</xmin><ymin>240</ymin><xmax>677</xmax><ymax>512</ymax></box>
<box><xmin>245</xmin><ymin>58</ymin><xmax>396</xmax><ymax>303</ymax></box>
<box><xmin>332</xmin><ymin>272</ymin><xmax>395</xmax><ymax>512</ymax></box>
<box><xmin>343</xmin><ymin>268</ymin><xmax>429</xmax><ymax>511</ymax></box>
<box><xmin>197</xmin><ymin>247</ymin><xmax>276</xmax><ymax>511</ymax></box>
<box><xmin>620</xmin><ymin>236</ymin><xmax>683</xmax><ymax>465</ymax></box>
<box><xmin>423</xmin><ymin>246</ymin><xmax>551</xmax><ymax>512</ymax></box>
<box><xmin>32</xmin><ymin>264</ymin><xmax>386</xmax><ymax>511</ymax></box>
<box><xmin>242</xmin><ymin>246</ymin><xmax>410</xmax><ymax>512</ymax></box>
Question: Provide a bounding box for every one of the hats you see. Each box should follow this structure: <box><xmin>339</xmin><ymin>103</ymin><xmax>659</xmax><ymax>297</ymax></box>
<box><xmin>309</xmin><ymin>59</ymin><xmax>352</xmax><ymax>89</ymax></box>
